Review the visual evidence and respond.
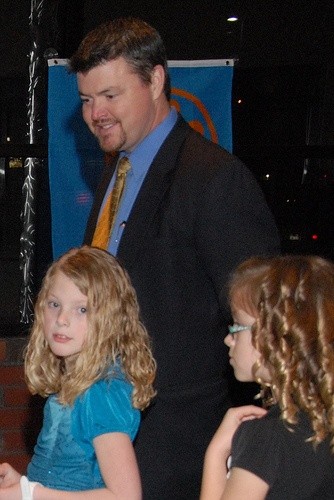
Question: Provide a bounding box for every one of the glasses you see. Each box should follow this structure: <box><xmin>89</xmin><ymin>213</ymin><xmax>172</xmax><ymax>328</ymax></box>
<box><xmin>229</xmin><ymin>324</ymin><xmax>254</xmax><ymax>341</ymax></box>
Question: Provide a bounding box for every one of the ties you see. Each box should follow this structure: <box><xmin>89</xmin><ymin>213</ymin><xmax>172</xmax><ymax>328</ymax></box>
<box><xmin>91</xmin><ymin>155</ymin><xmax>131</xmax><ymax>250</ymax></box>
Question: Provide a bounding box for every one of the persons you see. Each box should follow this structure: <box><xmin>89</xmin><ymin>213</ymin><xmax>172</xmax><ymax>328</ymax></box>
<box><xmin>196</xmin><ymin>255</ymin><xmax>334</xmax><ymax>500</ymax></box>
<box><xmin>0</xmin><ymin>243</ymin><xmax>159</xmax><ymax>500</ymax></box>
<box><xmin>68</xmin><ymin>18</ymin><xmax>283</xmax><ymax>499</ymax></box>
